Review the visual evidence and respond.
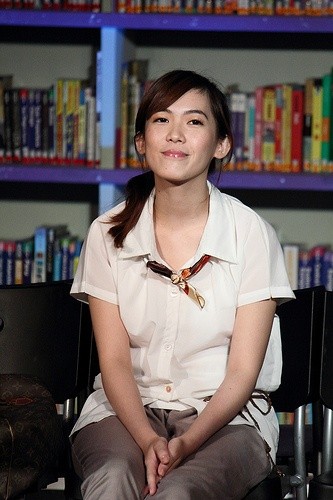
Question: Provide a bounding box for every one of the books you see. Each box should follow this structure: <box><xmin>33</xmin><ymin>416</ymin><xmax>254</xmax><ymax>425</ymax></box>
<box><xmin>119</xmin><ymin>59</ymin><xmax>333</xmax><ymax>173</ymax></box>
<box><xmin>1</xmin><ymin>0</ymin><xmax>103</xmax><ymax>13</ymax></box>
<box><xmin>116</xmin><ymin>1</ymin><xmax>333</xmax><ymax>16</ymax></box>
<box><xmin>57</xmin><ymin>397</ymin><xmax>79</xmax><ymax>423</ymax></box>
<box><xmin>281</xmin><ymin>243</ymin><xmax>333</xmax><ymax>291</ymax></box>
<box><xmin>0</xmin><ymin>50</ymin><xmax>102</xmax><ymax>168</ymax></box>
<box><xmin>276</xmin><ymin>402</ymin><xmax>313</xmax><ymax>425</ymax></box>
<box><xmin>0</xmin><ymin>224</ymin><xmax>84</xmax><ymax>285</ymax></box>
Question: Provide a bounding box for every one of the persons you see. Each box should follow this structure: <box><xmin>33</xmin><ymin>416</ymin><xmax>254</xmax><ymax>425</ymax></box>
<box><xmin>70</xmin><ymin>69</ymin><xmax>297</xmax><ymax>500</ymax></box>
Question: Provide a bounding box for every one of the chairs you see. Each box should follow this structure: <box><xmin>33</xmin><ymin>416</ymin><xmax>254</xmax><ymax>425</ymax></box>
<box><xmin>0</xmin><ymin>279</ymin><xmax>81</xmax><ymax>500</ymax></box>
<box><xmin>65</xmin><ymin>291</ymin><xmax>314</xmax><ymax>500</ymax></box>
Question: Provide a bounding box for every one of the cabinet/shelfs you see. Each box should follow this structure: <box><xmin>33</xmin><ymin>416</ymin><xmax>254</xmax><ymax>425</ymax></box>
<box><xmin>0</xmin><ymin>0</ymin><xmax>333</xmax><ymax>291</ymax></box>
